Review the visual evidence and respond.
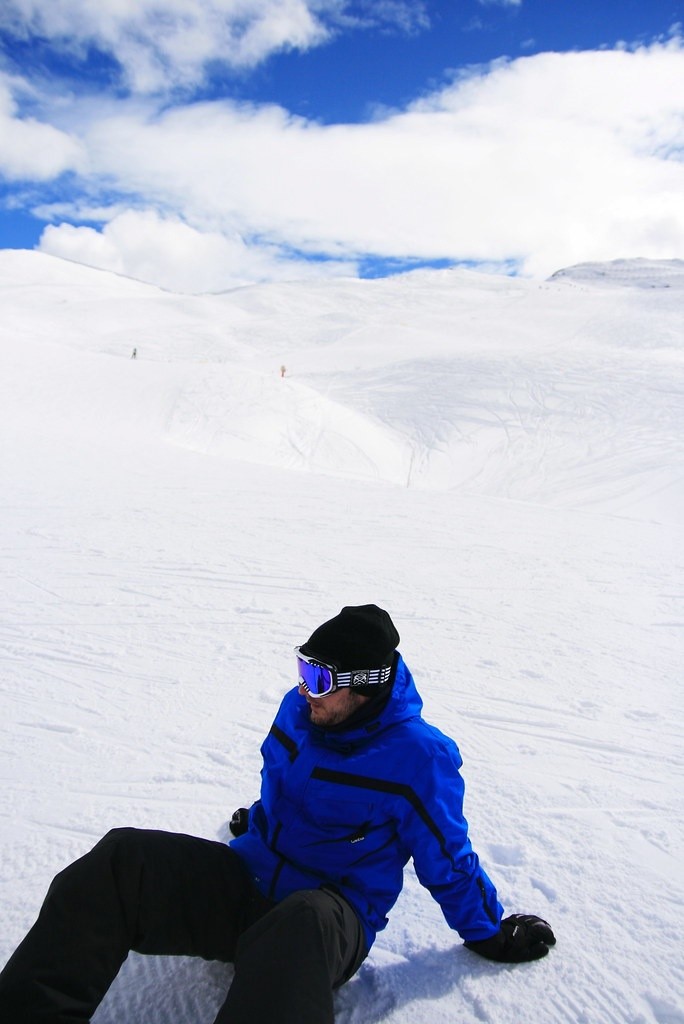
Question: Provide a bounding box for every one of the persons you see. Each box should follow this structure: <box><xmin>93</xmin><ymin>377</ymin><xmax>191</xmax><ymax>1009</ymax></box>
<box><xmin>0</xmin><ymin>605</ymin><xmax>556</xmax><ymax>1024</ymax></box>
<box><xmin>280</xmin><ymin>365</ymin><xmax>286</xmax><ymax>377</ymax></box>
<box><xmin>130</xmin><ymin>348</ymin><xmax>137</xmax><ymax>359</ymax></box>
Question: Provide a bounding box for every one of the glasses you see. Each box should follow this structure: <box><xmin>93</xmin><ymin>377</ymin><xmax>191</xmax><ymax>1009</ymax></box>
<box><xmin>296</xmin><ymin>648</ymin><xmax>339</xmax><ymax>697</ymax></box>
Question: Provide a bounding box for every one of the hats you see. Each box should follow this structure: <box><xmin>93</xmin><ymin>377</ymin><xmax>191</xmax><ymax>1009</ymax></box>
<box><xmin>298</xmin><ymin>604</ymin><xmax>399</xmax><ymax>700</ymax></box>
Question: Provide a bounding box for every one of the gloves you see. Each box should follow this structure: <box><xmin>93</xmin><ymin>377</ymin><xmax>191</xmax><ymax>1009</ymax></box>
<box><xmin>229</xmin><ymin>807</ymin><xmax>251</xmax><ymax>837</ymax></box>
<box><xmin>462</xmin><ymin>913</ymin><xmax>555</xmax><ymax>963</ymax></box>
